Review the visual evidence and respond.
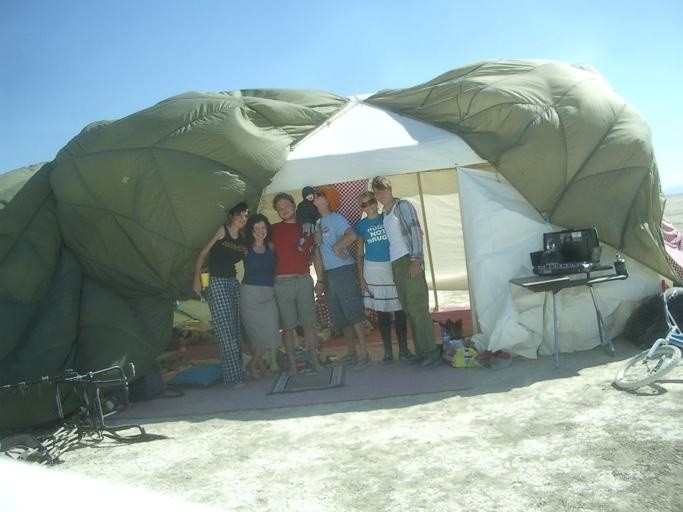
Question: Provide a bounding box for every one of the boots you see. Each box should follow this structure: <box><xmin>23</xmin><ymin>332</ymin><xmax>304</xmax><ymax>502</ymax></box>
<box><xmin>397</xmin><ymin>335</ymin><xmax>417</xmax><ymax>362</ymax></box>
<box><xmin>381</xmin><ymin>335</ymin><xmax>394</xmax><ymax>364</ymax></box>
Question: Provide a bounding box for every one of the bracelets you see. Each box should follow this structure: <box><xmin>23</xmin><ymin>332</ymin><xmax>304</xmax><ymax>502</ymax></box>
<box><xmin>317</xmin><ymin>281</ymin><xmax>324</xmax><ymax>284</ymax></box>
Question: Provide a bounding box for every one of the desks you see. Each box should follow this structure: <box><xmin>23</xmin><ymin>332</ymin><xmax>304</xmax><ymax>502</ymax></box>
<box><xmin>507</xmin><ymin>262</ymin><xmax>627</xmax><ymax>370</ymax></box>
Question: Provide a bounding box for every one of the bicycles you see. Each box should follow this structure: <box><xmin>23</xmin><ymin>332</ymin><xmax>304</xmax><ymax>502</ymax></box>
<box><xmin>616</xmin><ymin>286</ymin><xmax>682</xmax><ymax>391</ymax></box>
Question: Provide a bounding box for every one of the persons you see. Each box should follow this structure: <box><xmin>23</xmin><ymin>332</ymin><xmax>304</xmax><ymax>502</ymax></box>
<box><xmin>369</xmin><ymin>175</ymin><xmax>440</xmax><ymax>367</ymax></box>
<box><xmin>356</xmin><ymin>190</ymin><xmax>417</xmax><ymax>362</ymax></box>
<box><xmin>313</xmin><ymin>186</ymin><xmax>370</xmax><ymax>367</ymax></box>
<box><xmin>268</xmin><ymin>192</ymin><xmax>326</xmax><ymax>378</ymax></box>
<box><xmin>296</xmin><ymin>187</ymin><xmax>322</xmax><ymax>251</ymax></box>
<box><xmin>193</xmin><ymin>201</ymin><xmax>250</xmax><ymax>389</ymax></box>
<box><xmin>239</xmin><ymin>214</ymin><xmax>283</xmax><ymax>379</ymax></box>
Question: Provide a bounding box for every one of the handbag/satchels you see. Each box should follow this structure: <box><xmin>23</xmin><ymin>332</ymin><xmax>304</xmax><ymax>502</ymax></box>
<box><xmin>314</xmin><ymin>295</ymin><xmax>332</xmax><ymax>329</ymax></box>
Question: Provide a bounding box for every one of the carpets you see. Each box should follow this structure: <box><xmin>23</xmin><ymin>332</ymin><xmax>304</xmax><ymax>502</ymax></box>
<box><xmin>264</xmin><ymin>363</ymin><xmax>344</xmax><ymax>397</ymax></box>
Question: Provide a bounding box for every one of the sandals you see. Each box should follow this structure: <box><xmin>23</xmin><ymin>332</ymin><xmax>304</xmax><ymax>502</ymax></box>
<box><xmin>353</xmin><ymin>351</ymin><xmax>372</xmax><ymax>370</ymax></box>
<box><xmin>338</xmin><ymin>353</ymin><xmax>357</xmax><ymax>366</ymax></box>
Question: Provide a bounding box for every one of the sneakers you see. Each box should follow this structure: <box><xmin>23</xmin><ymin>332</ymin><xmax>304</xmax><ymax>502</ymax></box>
<box><xmin>407</xmin><ymin>354</ymin><xmax>442</xmax><ymax>370</ymax></box>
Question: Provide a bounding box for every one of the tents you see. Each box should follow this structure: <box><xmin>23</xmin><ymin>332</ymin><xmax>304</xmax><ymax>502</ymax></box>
<box><xmin>0</xmin><ymin>58</ymin><xmax>682</xmax><ymax>431</ymax></box>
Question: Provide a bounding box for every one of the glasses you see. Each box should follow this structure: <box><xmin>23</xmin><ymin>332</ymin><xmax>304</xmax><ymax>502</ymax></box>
<box><xmin>359</xmin><ymin>199</ymin><xmax>376</xmax><ymax>208</ymax></box>
